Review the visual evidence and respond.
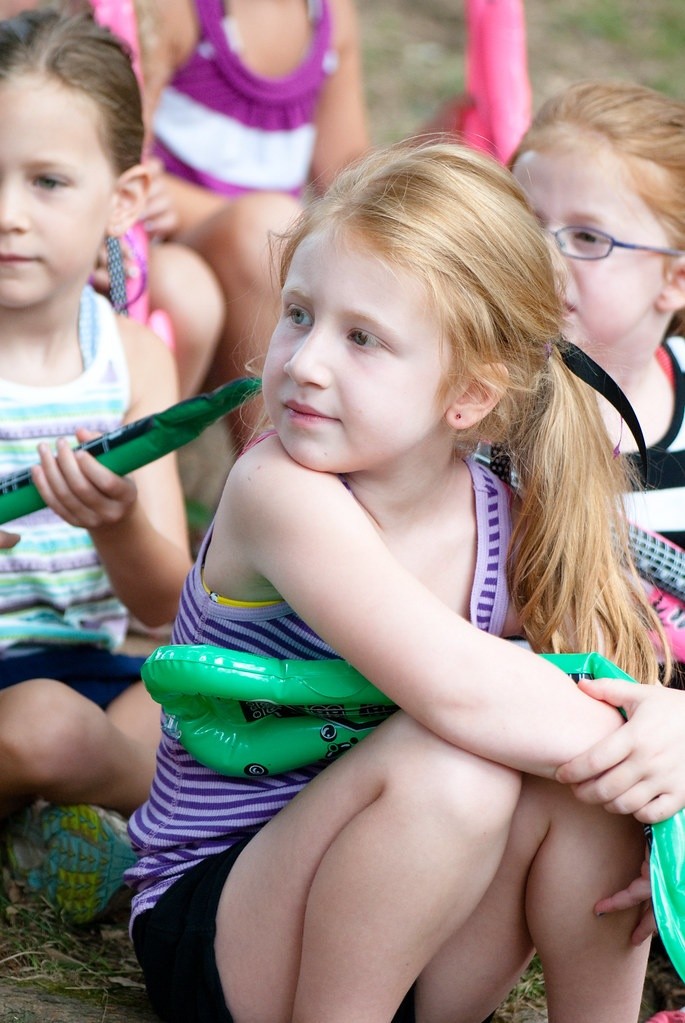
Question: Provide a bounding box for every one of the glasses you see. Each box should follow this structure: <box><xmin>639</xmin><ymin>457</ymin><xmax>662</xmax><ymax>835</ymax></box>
<box><xmin>542</xmin><ymin>226</ymin><xmax>685</xmax><ymax>261</ymax></box>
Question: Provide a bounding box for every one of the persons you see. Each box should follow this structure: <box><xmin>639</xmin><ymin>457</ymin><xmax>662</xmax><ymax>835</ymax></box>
<box><xmin>505</xmin><ymin>82</ymin><xmax>685</xmax><ymax>687</ymax></box>
<box><xmin>0</xmin><ymin>0</ymin><xmax>374</xmax><ymax>924</ymax></box>
<box><xmin>127</xmin><ymin>134</ymin><xmax>685</xmax><ymax>1023</ymax></box>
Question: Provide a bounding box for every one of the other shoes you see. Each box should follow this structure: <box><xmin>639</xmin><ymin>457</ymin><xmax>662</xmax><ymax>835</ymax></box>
<box><xmin>7</xmin><ymin>799</ymin><xmax>140</xmax><ymax>925</ymax></box>
